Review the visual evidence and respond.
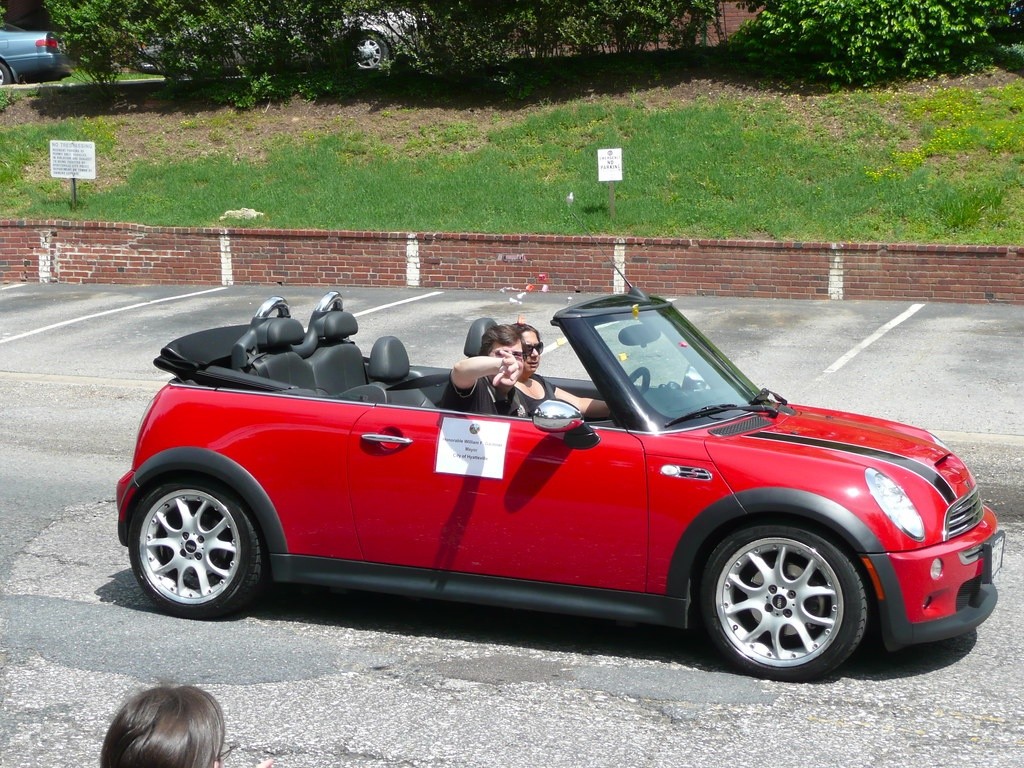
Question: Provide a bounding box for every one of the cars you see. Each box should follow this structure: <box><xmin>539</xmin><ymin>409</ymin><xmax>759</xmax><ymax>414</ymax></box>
<box><xmin>0</xmin><ymin>23</ymin><xmax>70</xmax><ymax>88</ymax></box>
<box><xmin>136</xmin><ymin>10</ymin><xmax>428</xmax><ymax>72</ymax></box>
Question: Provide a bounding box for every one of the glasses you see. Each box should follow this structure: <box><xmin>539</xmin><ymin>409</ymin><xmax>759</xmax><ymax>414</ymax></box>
<box><xmin>522</xmin><ymin>342</ymin><xmax>543</xmax><ymax>357</ymax></box>
<box><xmin>494</xmin><ymin>350</ymin><xmax>525</xmax><ymax>362</ymax></box>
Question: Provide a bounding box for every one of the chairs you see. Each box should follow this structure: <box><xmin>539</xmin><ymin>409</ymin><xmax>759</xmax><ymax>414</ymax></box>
<box><xmin>302</xmin><ymin>291</ymin><xmax>389</xmax><ymax>405</ymax></box>
<box><xmin>368</xmin><ymin>336</ymin><xmax>413</xmax><ymax>408</ymax></box>
<box><xmin>230</xmin><ymin>295</ymin><xmax>326</xmax><ymax>398</ymax></box>
<box><xmin>463</xmin><ymin>317</ymin><xmax>499</xmax><ymax>357</ymax></box>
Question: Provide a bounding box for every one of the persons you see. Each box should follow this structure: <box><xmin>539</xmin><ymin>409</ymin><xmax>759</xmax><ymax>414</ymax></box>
<box><xmin>440</xmin><ymin>321</ymin><xmax>615</xmax><ymax>419</ymax></box>
<box><xmin>100</xmin><ymin>685</ymin><xmax>230</xmax><ymax>768</ymax></box>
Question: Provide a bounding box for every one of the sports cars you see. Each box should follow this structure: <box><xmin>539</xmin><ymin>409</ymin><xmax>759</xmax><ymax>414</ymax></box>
<box><xmin>116</xmin><ymin>213</ymin><xmax>1005</xmax><ymax>682</ymax></box>
